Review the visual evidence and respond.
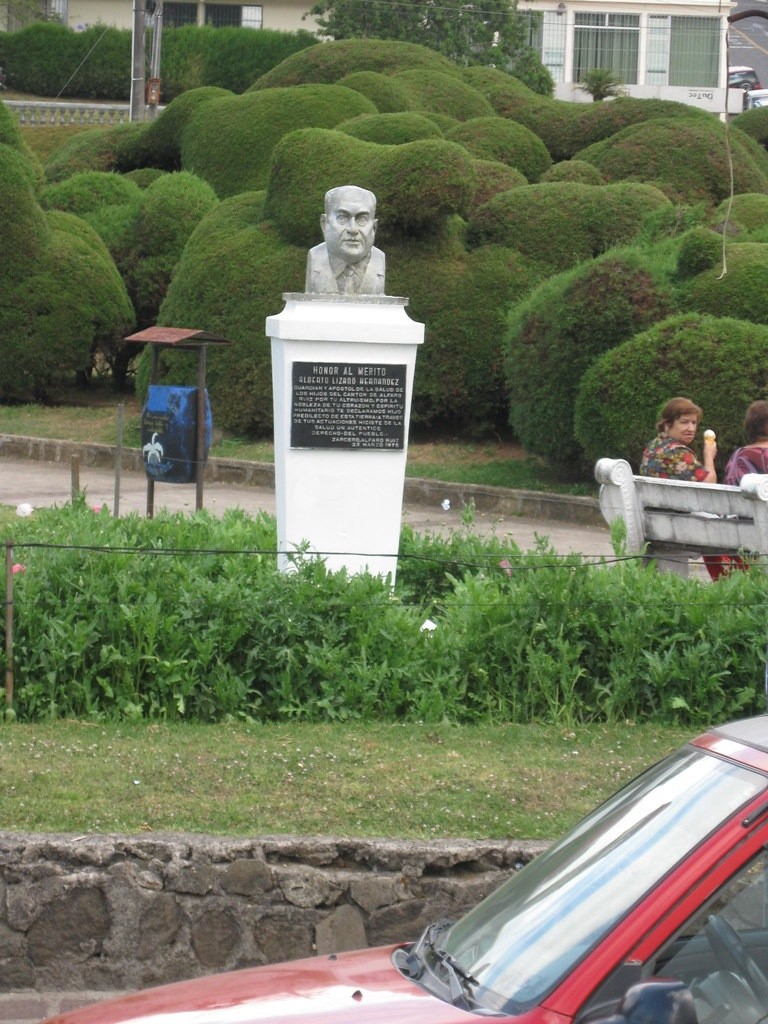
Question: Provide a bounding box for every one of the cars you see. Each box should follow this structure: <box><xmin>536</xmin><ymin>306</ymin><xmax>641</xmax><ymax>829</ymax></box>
<box><xmin>38</xmin><ymin>712</ymin><xmax>768</xmax><ymax>1024</ymax></box>
<box><xmin>729</xmin><ymin>66</ymin><xmax>761</xmax><ymax>91</ymax></box>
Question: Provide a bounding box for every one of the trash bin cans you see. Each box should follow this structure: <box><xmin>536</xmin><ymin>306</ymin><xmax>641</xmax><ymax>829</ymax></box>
<box><xmin>120</xmin><ymin>324</ymin><xmax>234</xmax><ymax>517</ymax></box>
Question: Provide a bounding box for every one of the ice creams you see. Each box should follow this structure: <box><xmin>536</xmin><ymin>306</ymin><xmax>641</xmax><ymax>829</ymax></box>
<box><xmin>703</xmin><ymin>429</ymin><xmax>716</xmax><ymax>446</ymax></box>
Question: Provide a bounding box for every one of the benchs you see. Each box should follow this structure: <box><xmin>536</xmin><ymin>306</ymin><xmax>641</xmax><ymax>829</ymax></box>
<box><xmin>594</xmin><ymin>457</ymin><xmax>768</xmax><ymax>578</ymax></box>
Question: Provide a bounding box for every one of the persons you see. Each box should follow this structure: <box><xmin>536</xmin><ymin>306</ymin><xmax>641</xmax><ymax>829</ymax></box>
<box><xmin>725</xmin><ymin>400</ymin><xmax>768</xmax><ymax>486</ymax></box>
<box><xmin>639</xmin><ymin>397</ymin><xmax>749</xmax><ymax>582</ymax></box>
<box><xmin>305</xmin><ymin>185</ymin><xmax>386</xmax><ymax>294</ymax></box>
